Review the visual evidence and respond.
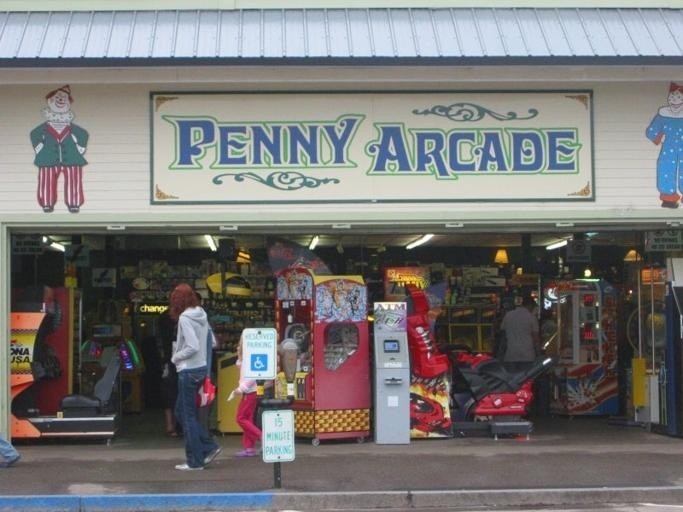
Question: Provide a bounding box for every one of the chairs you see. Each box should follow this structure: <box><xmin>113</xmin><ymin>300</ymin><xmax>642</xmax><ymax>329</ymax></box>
<box><xmin>59</xmin><ymin>346</ymin><xmax>128</xmax><ymax>417</ymax></box>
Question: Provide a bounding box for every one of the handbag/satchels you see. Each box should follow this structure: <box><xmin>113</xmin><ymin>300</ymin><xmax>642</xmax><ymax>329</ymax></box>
<box><xmin>194</xmin><ymin>375</ymin><xmax>216</xmax><ymax>408</ymax></box>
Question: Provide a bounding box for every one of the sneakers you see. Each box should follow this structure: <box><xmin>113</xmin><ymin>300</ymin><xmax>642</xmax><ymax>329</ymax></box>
<box><xmin>204</xmin><ymin>446</ymin><xmax>223</xmax><ymax>464</ymax></box>
<box><xmin>235</xmin><ymin>450</ymin><xmax>255</xmax><ymax>456</ymax></box>
<box><xmin>164</xmin><ymin>422</ymin><xmax>184</xmax><ymax>440</ymax></box>
<box><xmin>174</xmin><ymin>464</ymin><xmax>203</xmax><ymax>471</ymax></box>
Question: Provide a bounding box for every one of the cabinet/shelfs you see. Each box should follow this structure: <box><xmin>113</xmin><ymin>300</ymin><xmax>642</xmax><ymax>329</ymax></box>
<box><xmin>199</xmin><ymin>297</ymin><xmax>279</xmax><ymax>356</ymax></box>
<box><xmin>432</xmin><ymin>300</ymin><xmax>502</xmax><ymax>357</ymax></box>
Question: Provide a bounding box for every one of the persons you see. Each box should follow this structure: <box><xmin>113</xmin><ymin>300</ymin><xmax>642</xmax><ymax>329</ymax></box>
<box><xmin>500</xmin><ymin>297</ymin><xmax>541</xmax><ymax>373</ymax></box>
<box><xmin>1</xmin><ymin>440</ymin><xmax>20</xmax><ymax>467</ymax></box>
<box><xmin>541</xmin><ymin>309</ymin><xmax>557</xmax><ymax>336</ymax></box>
<box><xmin>154</xmin><ymin>310</ymin><xmax>179</xmax><ymax>439</ymax></box>
<box><xmin>171</xmin><ymin>284</ymin><xmax>222</xmax><ymax>472</ymax></box>
<box><xmin>235</xmin><ymin>337</ymin><xmax>262</xmax><ymax>456</ymax></box>
